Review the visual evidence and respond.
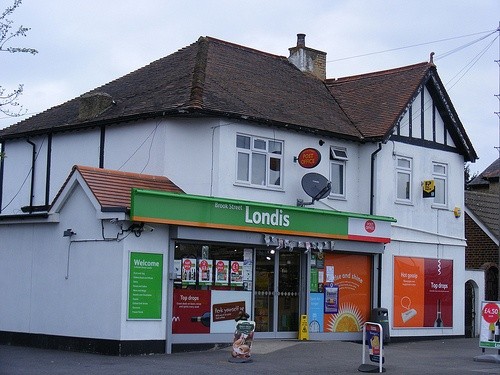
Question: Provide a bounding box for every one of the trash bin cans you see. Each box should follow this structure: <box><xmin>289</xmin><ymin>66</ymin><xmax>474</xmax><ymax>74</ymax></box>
<box><xmin>372</xmin><ymin>307</ymin><xmax>389</xmax><ymax>345</ymax></box>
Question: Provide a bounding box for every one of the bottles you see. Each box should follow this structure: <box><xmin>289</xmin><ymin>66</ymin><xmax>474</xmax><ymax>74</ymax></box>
<box><xmin>495</xmin><ymin>320</ymin><xmax>500</xmax><ymax>342</ymax></box>
<box><xmin>488</xmin><ymin>323</ymin><xmax>495</xmax><ymax>341</ymax></box>
<box><xmin>191</xmin><ymin>311</ymin><xmax>210</xmax><ymax>327</ymax></box>
